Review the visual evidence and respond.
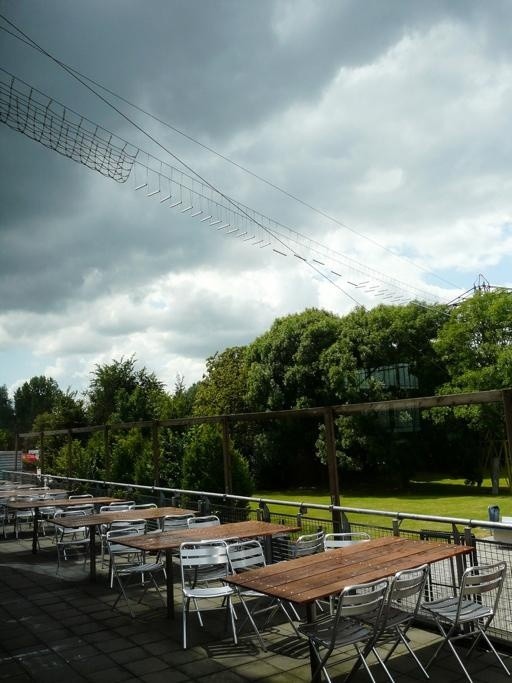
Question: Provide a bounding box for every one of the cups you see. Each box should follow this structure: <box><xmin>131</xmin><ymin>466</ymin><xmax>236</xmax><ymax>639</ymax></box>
<box><xmin>487</xmin><ymin>505</ymin><xmax>499</xmax><ymax>522</ymax></box>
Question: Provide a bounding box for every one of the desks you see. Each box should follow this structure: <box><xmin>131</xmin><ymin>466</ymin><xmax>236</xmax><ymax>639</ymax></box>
<box><xmin>221</xmin><ymin>534</ymin><xmax>474</xmax><ymax>680</ymax></box>
<box><xmin>107</xmin><ymin>520</ymin><xmax>301</xmax><ymax>635</ymax></box>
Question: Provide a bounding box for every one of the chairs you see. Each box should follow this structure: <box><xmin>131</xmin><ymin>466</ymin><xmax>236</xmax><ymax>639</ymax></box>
<box><xmin>346</xmin><ymin>564</ymin><xmax>430</xmax><ymax>681</ymax></box>
<box><xmin>265</xmin><ymin>531</ymin><xmax>379</xmax><ymax>619</ymax></box>
<box><xmin>0</xmin><ymin>478</ymin><xmax>242</xmax><ymax>626</ymax></box>
<box><xmin>421</xmin><ymin>561</ymin><xmax>509</xmax><ymax>681</ymax></box>
<box><xmin>226</xmin><ymin>539</ymin><xmax>301</xmax><ymax>652</ymax></box>
<box><xmin>180</xmin><ymin>540</ymin><xmax>239</xmax><ymax>650</ymax></box>
<box><xmin>298</xmin><ymin>578</ymin><xmax>390</xmax><ymax>680</ymax></box>
